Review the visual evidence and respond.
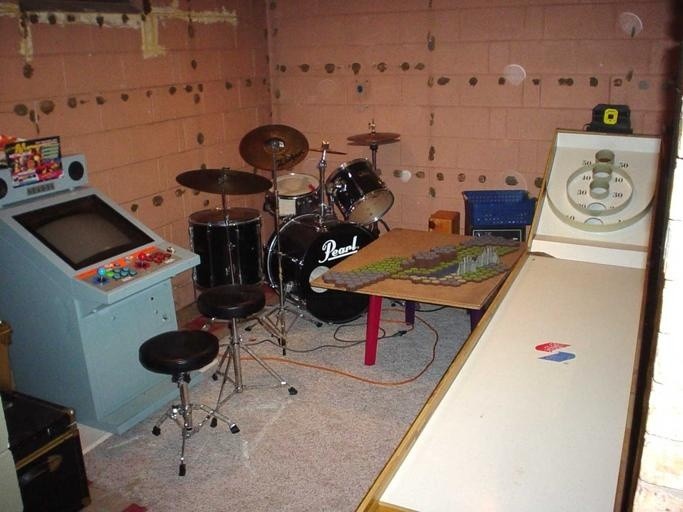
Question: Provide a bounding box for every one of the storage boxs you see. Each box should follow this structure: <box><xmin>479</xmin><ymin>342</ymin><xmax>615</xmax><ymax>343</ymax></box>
<box><xmin>0</xmin><ymin>388</ymin><xmax>91</xmax><ymax>511</ymax></box>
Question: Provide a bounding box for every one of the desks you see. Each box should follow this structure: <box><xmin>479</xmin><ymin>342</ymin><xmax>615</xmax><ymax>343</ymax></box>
<box><xmin>309</xmin><ymin>226</ymin><xmax>527</xmax><ymax>367</ymax></box>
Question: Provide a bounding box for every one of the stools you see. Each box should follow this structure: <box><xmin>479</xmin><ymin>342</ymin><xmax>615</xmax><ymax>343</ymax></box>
<box><xmin>136</xmin><ymin>329</ymin><xmax>240</xmax><ymax>479</ymax></box>
<box><xmin>196</xmin><ymin>284</ymin><xmax>300</xmax><ymax>427</ymax></box>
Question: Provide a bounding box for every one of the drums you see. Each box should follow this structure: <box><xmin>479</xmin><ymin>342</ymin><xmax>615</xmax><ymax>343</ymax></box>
<box><xmin>268</xmin><ymin>173</ymin><xmax>322</xmax><ymax>217</ymax></box>
<box><xmin>327</xmin><ymin>157</ymin><xmax>394</xmax><ymax>226</ymax></box>
<box><xmin>189</xmin><ymin>207</ymin><xmax>264</xmax><ymax>290</ymax></box>
<box><xmin>263</xmin><ymin>212</ymin><xmax>377</xmax><ymax>324</ymax></box>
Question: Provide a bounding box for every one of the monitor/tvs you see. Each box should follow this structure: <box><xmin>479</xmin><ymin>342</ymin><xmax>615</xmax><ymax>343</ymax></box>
<box><xmin>12</xmin><ymin>194</ymin><xmax>155</xmax><ymax>270</ymax></box>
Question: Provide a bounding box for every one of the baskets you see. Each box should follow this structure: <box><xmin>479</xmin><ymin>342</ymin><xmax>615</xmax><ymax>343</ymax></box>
<box><xmin>462</xmin><ymin>188</ymin><xmax>535</xmax><ymax>227</ymax></box>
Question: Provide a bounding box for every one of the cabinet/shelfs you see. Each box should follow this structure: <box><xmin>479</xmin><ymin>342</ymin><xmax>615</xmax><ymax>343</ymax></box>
<box><xmin>0</xmin><ymin>319</ymin><xmax>13</xmax><ymax>389</ymax></box>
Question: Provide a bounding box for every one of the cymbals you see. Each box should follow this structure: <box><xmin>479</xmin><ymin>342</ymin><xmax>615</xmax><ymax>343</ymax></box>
<box><xmin>239</xmin><ymin>124</ymin><xmax>309</xmax><ymax>170</ymax></box>
<box><xmin>309</xmin><ymin>148</ymin><xmax>347</xmax><ymax>155</ymax></box>
<box><xmin>348</xmin><ymin>131</ymin><xmax>401</xmax><ymax>146</ymax></box>
<box><xmin>175</xmin><ymin>169</ymin><xmax>272</xmax><ymax>194</ymax></box>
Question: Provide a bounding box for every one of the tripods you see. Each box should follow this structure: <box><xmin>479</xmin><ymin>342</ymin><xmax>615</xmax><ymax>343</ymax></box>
<box><xmin>244</xmin><ymin>146</ymin><xmax>323</xmax><ymax>351</ymax></box>
